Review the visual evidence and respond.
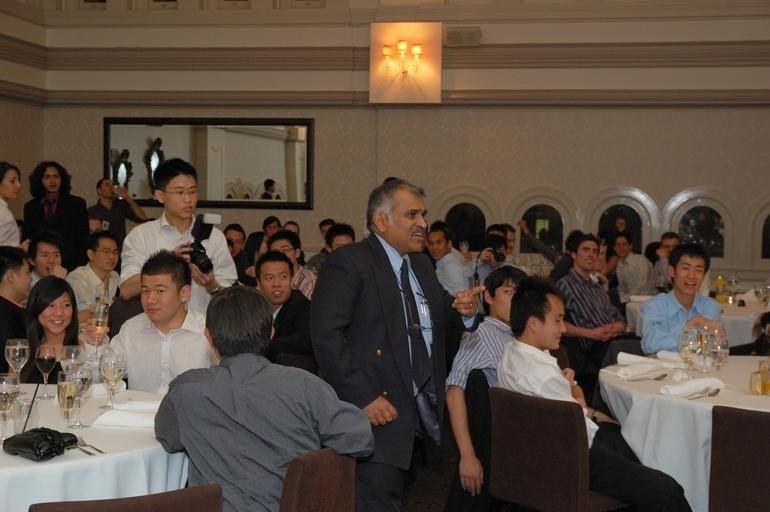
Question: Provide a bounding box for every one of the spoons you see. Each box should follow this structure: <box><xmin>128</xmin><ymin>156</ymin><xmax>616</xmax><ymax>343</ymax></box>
<box><xmin>629</xmin><ymin>373</ymin><xmax>667</xmax><ymax>381</ymax></box>
<box><xmin>689</xmin><ymin>388</ymin><xmax>720</xmax><ymax>401</ymax></box>
<box><xmin>71</xmin><ymin>444</ymin><xmax>96</xmax><ymax>456</ymax></box>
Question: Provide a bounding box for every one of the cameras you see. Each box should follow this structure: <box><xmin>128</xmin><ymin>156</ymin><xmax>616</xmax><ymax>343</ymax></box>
<box><xmin>181</xmin><ymin>213</ymin><xmax>221</xmax><ymax>273</ymax></box>
<box><xmin>467</xmin><ymin>233</ymin><xmax>506</xmax><ymax>262</ymax></box>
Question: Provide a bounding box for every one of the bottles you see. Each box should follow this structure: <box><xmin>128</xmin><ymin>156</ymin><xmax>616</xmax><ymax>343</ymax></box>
<box><xmin>751</xmin><ymin>361</ymin><xmax>770</xmax><ymax>395</ymax></box>
<box><xmin>715</xmin><ymin>275</ymin><xmax>725</xmax><ymax>303</ymax></box>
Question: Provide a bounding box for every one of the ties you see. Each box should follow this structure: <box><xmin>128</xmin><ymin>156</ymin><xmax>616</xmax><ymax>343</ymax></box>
<box><xmin>401</xmin><ymin>258</ymin><xmax>437</xmax><ymax>395</ymax></box>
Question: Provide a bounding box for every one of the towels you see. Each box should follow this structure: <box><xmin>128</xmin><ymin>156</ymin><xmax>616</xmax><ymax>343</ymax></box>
<box><xmin>617</xmin><ymin>351</ymin><xmax>685</xmax><ymax>370</ymax></box>
<box><xmin>656</xmin><ymin>350</ymin><xmax>712</xmax><ymax>367</ymax></box>
<box><xmin>617</xmin><ymin>362</ymin><xmax>666</xmax><ymax>381</ymax></box>
<box><xmin>660</xmin><ymin>377</ymin><xmax>726</xmax><ymax>396</ymax></box>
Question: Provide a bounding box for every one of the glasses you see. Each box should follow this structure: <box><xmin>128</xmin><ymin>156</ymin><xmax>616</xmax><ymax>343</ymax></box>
<box><xmin>94</xmin><ymin>249</ymin><xmax>119</xmax><ymax>255</ymax></box>
<box><xmin>282</xmin><ymin>248</ymin><xmax>295</xmax><ymax>252</ymax></box>
<box><xmin>161</xmin><ymin>190</ymin><xmax>197</xmax><ymax>196</ymax></box>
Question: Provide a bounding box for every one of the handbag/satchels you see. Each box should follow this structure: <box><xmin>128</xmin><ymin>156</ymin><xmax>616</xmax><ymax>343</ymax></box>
<box><xmin>2</xmin><ymin>427</ymin><xmax>78</xmax><ymax>462</ymax></box>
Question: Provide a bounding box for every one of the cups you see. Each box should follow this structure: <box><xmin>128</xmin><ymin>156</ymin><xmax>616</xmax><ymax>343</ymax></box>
<box><xmin>99</xmin><ymin>353</ymin><xmax>125</xmax><ymax>410</ymax></box>
<box><xmin>35</xmin><ymin>346</ymin><xmax>57</xmax><ymax>400</ymax></box>
<box><xmin>728</xmin><ymin>274</ymin><xmax>742</xmax><ymax>303</ymax></box>
<box><xmin>14</xmin><ymin>398</ymin><xmax>38</xmax><ymax>438</ymax></box>
<box><xmin>57</xmin><ymin>371</ymin><xmax>77</xmax><ymax>433</ymax></box>
<box><xmin>0</xmin><ymin>373</ymin><xmax>20</xmax><ymax>444</ymax></box>
<box><xmin>4</xmin><ymin>339</ymin><xmax>30</xmax><ymax>395</ymax></box>
<box><xmin>60</xmin><ymin>346</ymin><xmax>80</xmax><ymax>372</ymax></box>
<box><xmin>749</xmin><ymin>361</ymin><xmax>770</xmax><ymax>397</ymax></box>
<box><xmin>709</xmin><ymin>332</ymin><xmax>729</xmax><ymax>380</ymax></box>
<box><xmin>64</xmin><ymin>361</ymin><xmax>93</xmax><ymax>429</ymax></box>
<box><xmin>755</xmin><ymin>286</ymin><xmax>765</xmax><ymax>305</ymax></box>
<box><xmin>766</xmin><ymin>284</ymin><xmax>770</xmax><ymax>306</ymax></box>
<box><xmin>114</xmin><ymin>185</ymin><xmax>123</xmax><ymax>199</ymax></box>
<box><xmin>92</xmin><ymin>303</ymin><xmax>109</xmax><ymax>359</ymax></box>
<box><xmin>699</xmin><ymin>326</ymin><xmax>714</xmax><ymax>373</ymax></box>
<box><xmin>678</xmin><ymin>328</ymin><xmax>700</xmax><ymax>379</ymax></box>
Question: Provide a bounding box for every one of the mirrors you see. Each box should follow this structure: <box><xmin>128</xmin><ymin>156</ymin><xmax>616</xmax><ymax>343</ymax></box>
<box><xmin>102</xmin><ymin>117</ymin><xmax>312</xmax><ymax>208</ymax></box>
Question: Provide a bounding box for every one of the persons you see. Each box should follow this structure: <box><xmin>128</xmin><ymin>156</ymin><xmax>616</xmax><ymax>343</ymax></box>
<box><xmin>66</xmin><ymin>231</ymin><xmax>120</xmax><ymax>324</ymax></box>
<box><xmin>0</xmin><ymin>161</ymin><xmax>22</xmax><ymax>247</ymax></box>
<box><xmin>613</xmin><ymin>233</ymin><xmax>662</xmax><ymax>303</ymax></box>
<box><xmin>319</xmin><ymin>219</ymin><xmax>336</xmax><ymax>238</ymax></box>
<box><xmin>1</xmin><ymin>275</ymin><xmax>80</xmax><ymax>385</ymax></box>
<box><xmin>445</xmin><ymin>265</ymin><xmax>551</xmax><ymax>497</ymax></box>
<box><xmin>517</xmin><ymin>217</ymin><xmax>561</xmax><ymax>263</ymax></box>
<box><xmin>580</xmin><ymin>239</ymin><xmax>602</xmax><ymax>261</ymax></box>
<box><xmin>155</xmin><ymin>285</ymin><xmax>373</xmax><ymax>511</ymax></box>
<box><xmin>89</xmin><ymin>218</ymin><xmax>102</xmax><ymax>236</ymax></box>
<box><xmin>501</xmin><ymin>224</ymin><xmax>516</xmax><ymax>262</ymax></box>
<box><xmin>255</xmin><ymin>249</ymin><xmax>317</xmax><ymax>373</ymax></box>
<box><xmin>24</xmin><ymin>162</ymin><xmax>87</xmax><ymax>272</ymax></box>
<box><xmin>88</xmin><ymin>177</ymin><xmax>147</xmax><ymax>242</ymax></box>
<box><xmin>590</xmin><ymin>238</ymin><xmax>609</xmax><ymax>292</ymax></box>
<box><xmin>324</xmin><ymin>223</ymin><xmax>355</xmax><ymax>253</ymax></box>
<box><xmin>497</xmin><ymin>275</ymin><xmax>692</xmax><ymax>512</ymax></box>
<box><xmin>0</xmin><ymin>246</ymin><xmax>32</xmax><ymax>373</ymax></box>
<box><xmin>77</xmin><ymin>250</ymin><xmax>221</xmax><ymax>396</ymax></box>
<box><xmin>119</xmin><ymin>158</ymin><xmax>237</xmax><ymax>335</ymax></box>
<box><xmin>223</xmin><ymin>223</ymin><xmax>246</xmax><ymax>257</ymax></box>
<box><xmin>267</xmin><ymin>229</ymin><xmax>318</xmax><ymax>300</ymax></box>
<box><xmin>476</xmin><ymin>224</ymin><xmax>527</xmax><ymax>304</ymax></box>
<box><xmin>309</xmin><ymin>176</ymin><xmax>485</xmax><ymax>512</ymax></box>
<box><xmin>427</xmin><ymin>230</ymin><xmax>476</xmax><ymax>298</ymax></box>
<box><xmin>23</xmin><ymin>233</ymin><xmax>68</xmax><ymax>310</ymax></box>
<box><xmin>234</xmin><ymin>231</ymin><xmax>270</xmax><ymax>287</ymax></box>
<box><xmin>259</xmin><ymin>178</ymin><xmax>281</xmax><ymax>200</ymax></box>
<box><xmin>641</xmin><ymin>244</ymin><xmax>726</xmax><ymax>355</ymax></box>
<box><xmin>661</xmin><ymin>232</ymin><xmax>681</xmax><ymax>253</ymax></box>
<box><xmin>283</xmin><ymin>221</ymin><xmax>304</xmax><ymax>267</ymax></box>
<box><xmin>553</xmin><ymin>233</ymin><xmax>640</xmax><ymax>363</ymax></box>
<box><xmin>263</xmin><ymin>216</ymin><xmax>283</xmax><ymax>239</ymax></box>
<box><xmin>645</xmin><ymin>241</ymin><xmax>674</xmax><ymax>288</ymax></box>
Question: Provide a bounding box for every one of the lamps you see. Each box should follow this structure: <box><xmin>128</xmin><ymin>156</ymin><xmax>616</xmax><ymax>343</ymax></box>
<box><xmin>369</xmin><ymin>22</ymin><xmax>442</xmax><ymax>105</ymax></box>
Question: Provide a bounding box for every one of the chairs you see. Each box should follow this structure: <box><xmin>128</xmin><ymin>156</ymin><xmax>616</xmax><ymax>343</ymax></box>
<box><xmin>487</xmin><ymin>387</ymin><xmax>625</xmax><ymax>512</ymax></box>
<box><xmin>710</xmin><ymin>405</ymin><xmax>770</xmax><ymax>512</ymax></box>
<box><xmin>27</xmin><ymin>484</ymin><xmax>224</xmax><ymax>512</ymax></box>
<box><xmin>280</xmin><ymin>454</ymin><xmax>357</xmax><ymax>512</ymax></box>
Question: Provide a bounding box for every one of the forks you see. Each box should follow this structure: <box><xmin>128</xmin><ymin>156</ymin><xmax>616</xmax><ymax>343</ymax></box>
<box><xmin>683</xmin><ymin>387</ymin><xmax>711</xmax><ymax>398</ymax></box>
<box><xmin>78</xmin><ymin>436</ymin><xmax>104</xmax><ymax>454</ymax></box>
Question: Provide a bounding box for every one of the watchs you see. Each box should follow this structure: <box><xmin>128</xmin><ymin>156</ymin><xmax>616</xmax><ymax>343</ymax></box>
<box><xmin>210</xmin><ymin>284</ymin><xmax>220</xmax><ymax>295</ymax></box>
